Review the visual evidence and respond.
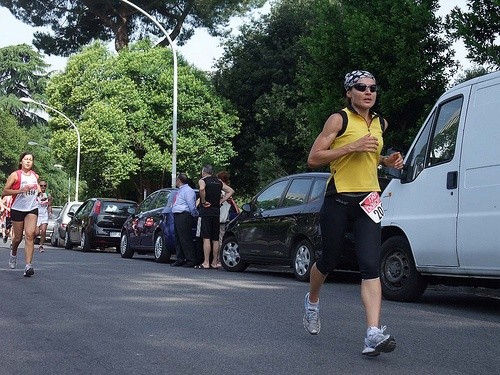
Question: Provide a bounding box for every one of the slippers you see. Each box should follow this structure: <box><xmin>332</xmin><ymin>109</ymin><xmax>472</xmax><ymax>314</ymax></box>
<box><xmin>210</xmin><ymin>264</ymin><xmax>220</xmax><ymax>269</ymax></box>
<box><xmin>194</xmin><ymin>264</ymin><xmax>210</xmax><ymax>269</ymax></box>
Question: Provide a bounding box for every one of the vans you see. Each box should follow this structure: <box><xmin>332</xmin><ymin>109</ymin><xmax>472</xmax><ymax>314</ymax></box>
<box><xmin>380</xmin><ymin>72</ymin><xmax>500</xmax><ymax>302</ymax></box>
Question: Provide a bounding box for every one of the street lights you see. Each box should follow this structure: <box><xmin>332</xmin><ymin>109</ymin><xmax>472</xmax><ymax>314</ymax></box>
<box><xmin>27</xmin><ymin>141</ymin><xmax>71</xmax><ymax>201</ymax></box>
<box><xmin>19</xmin><ymin>97</ymin><xmax>81</xmax><ymax>201</ymax></box>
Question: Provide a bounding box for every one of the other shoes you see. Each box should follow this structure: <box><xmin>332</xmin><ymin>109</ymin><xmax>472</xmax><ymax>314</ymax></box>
<box><xmin>9</xmin><ymin>244</ymin><xmax>16</xmax><ymax>250</ymax></box>
<box><xmin>170</xmin><ymin>261</ymin><xmax>183</xmax><ymax>267</ymax></box>
<box><xmin>3</xmin><ymin>231</ymin><xmax>7</xmax><ymax>243</ymax></box>
<box><xmin>39</xmin><ymin>246</ymin><xmax>44</xmax><ymax>252</ymax></box>
<box><xmin>184</xmin><ymin>261</ymin><xmax>195</xmax><ymax>268</ymax></box>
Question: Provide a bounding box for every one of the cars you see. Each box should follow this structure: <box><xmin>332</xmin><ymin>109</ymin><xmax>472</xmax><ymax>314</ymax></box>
<box><xmin>34</xmin><ymin>198</ymin><xmax>139</xmax><ymax>253</ymax></box>
<box><xmin>220</xmin><ymin>173</ymin><xmax>332</xmax><ymax>282</ymax></box>
<box><xmin>120</xmin><ymin>188</ymin><xmax>240</xmax><ymax>263</ymax></box>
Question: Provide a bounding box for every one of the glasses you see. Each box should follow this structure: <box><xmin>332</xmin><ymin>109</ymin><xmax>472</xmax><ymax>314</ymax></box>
<box><xmin>40</xmin><ymin>185</ymin><xmax>47</xmax><ymax>187</ymax></box>
<box><xmin>352</xmin><ymin>82</ymin><xmax>377</xmax><ymax>93</ymax></box>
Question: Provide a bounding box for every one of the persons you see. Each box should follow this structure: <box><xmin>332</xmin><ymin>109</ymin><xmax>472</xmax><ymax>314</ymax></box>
<box><xmin>35</xmin><ymin>180</ymin><xmax>53</xmax><ymax>253</ymax></box>
<box><xmin>303</xmin><ymin>71</ymin><xmax>404</xmax><ymax>356</ymax></box>
<box><xmin>170</xmin><ymin>173</ymin><xmax>198</xmax><ymax>268</ymax></box>
<box><xmin>2</xmin><ymin>152</ymin><xmax>43</xmax><ymax>276</ymax></box>
<box><xmin>0</xmin><ymin>195</ymin><xmax>15</xmax><ymax>245</ymax></box>
<box><xmin>194</xmin><ymin>165</ymin><xmax>234</xmax><ymax>269</ymax></box>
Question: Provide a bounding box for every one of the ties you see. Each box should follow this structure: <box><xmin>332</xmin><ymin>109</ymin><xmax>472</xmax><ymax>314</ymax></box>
<box><xmin>172</xmin><ymin>189</ymin><xmax>180</xmax><ymax>204</ymax></box>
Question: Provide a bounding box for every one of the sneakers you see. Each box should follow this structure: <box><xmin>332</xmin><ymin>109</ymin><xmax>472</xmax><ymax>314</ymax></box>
<box><xmin>362</xmin><ymin>324</ymin><xmax>397</xmax><ymax>357</ymax></box>
<box><xmin>9</xmin><ymin>249</ymin><xmax>17</xmax><ymax>269</ymax></box>
<box><xmin>23</xmin><ymin>264</ymin><xmax>35</xmax><ymax>277</ymax></box>
<box><xmin>303</xmin><ymin>292</ymin><xmax>322</xmax><ymax>335</ymax></box>
